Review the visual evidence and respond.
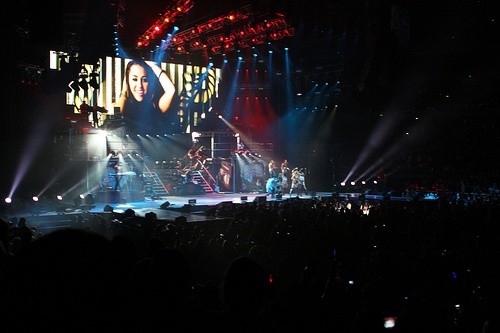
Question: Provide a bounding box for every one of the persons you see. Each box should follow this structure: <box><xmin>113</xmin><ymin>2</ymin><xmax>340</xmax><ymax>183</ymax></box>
<box><xmin>187</xmin><ymin>145</ymin><xmax>196</xmax><ymax>169</ymax></box>
<box><xmin>268</xmin><ymin>159</ymin><xmax>277</xmax><ymax>177</ymax></box>
<box><xmin>297</xmin><ymin>171</ymin><xmax>309</xmax><ymax>195</ymax></box>
<box><xmin>0</xmin><ymin>181</ymin><xmax>500</xmax><ymax>333</ymax></box>
<box><xmin>288</xmin><ymin>167</ymin><xmax>302</xmax><ymax>198</ymax></box>
<box><xmin>280</xmin><ymin>159</ymin><xmax>290</xmax><ymax>188</ymax></box>
<box><xmin>195</xmin><ymin>146</ymin><xmax>206</xmax><ymax>169</ymax></box>
<box><xmin>113</xmin><ymin>60</ymin><xmax>176</xmax><ymax>129</ymax></box>
